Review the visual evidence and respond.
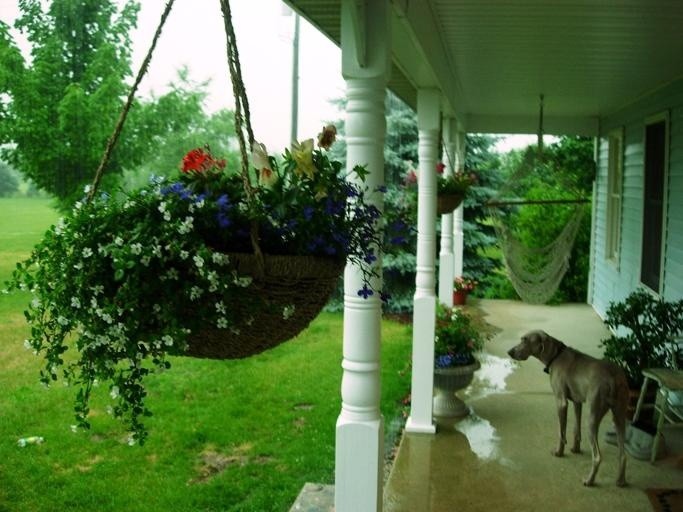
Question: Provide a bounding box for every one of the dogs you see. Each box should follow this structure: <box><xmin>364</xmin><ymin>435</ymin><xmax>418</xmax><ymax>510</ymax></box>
<box><xmin>506</xmin><ymin>329</ymin><xmax>630</xmax><ymax>487</ymax></box>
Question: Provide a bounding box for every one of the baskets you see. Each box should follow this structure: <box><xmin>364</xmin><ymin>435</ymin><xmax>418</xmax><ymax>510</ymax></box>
<box><xmin>100</xmin><ymin>251</ymin><xmax>344</xmax><ymax>358</ymax></box>
<box><xmin>419</xmin><ymin>191</ymin><xmax>465</xmax><ymax>216</ymax></box>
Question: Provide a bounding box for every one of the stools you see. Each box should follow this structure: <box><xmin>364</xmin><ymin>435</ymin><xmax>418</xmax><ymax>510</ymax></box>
<box><xmin>631</xmin><ymin>369</ymin><xmax>683</xmax><ymax>465</ymax></box>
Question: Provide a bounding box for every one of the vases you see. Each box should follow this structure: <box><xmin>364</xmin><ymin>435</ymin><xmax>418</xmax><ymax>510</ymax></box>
<box><xmin>453</xmin><ymin>291</ymin><xmax>468</xmax><ymax>305</ymax></box>
<box><xmin>433</xmin><ymin>360</ymin><xmax>481</xmax><ymax>417</ymax></box>
<box><xmin>437</xmin><ymin>189</ymin><xmax>466</xmax><ymax>214</ymax></box>
<box><xmin>140</xmin><ymin>247</ymin><xmax>346</xmax><ymax>361</ymax></box>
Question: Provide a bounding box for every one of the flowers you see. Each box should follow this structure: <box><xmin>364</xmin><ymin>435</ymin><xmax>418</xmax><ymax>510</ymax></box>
<box><xmin>435</xmin><ymin>298</ymin><xmax>497</xmax><ymax>369</ymax></box>
<box><xmin>453</xmin><ymin>276</ymin><xmax>479</xmax><ymax>294</ymax></box>
<box><xmin>0</xmin><ymin>124</ymin><xmax>420</xmax><ymax>448</ymax></box>
<box><xmin>397</xmin><ymin>163</ymin><xmax>479</xmax><ymax>221</ymax></box>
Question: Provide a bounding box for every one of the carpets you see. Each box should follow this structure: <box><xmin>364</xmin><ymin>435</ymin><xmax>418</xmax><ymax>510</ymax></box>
<box><xmin>645</xmin><ymin>487</ymin><xmax>683</xmax><ymax>512</ymax></box>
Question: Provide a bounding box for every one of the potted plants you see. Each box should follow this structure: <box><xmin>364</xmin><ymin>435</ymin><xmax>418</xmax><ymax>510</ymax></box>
<box><xmin>595</xmin><ymin>287</ymin><xmax>683</xmax><ymax>424</ymax></box>
